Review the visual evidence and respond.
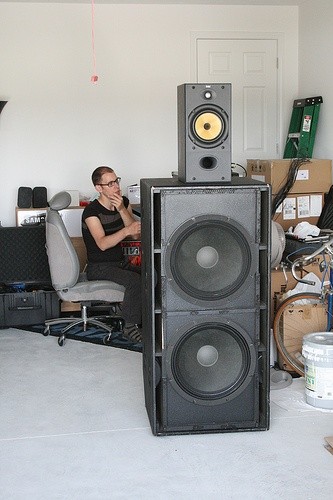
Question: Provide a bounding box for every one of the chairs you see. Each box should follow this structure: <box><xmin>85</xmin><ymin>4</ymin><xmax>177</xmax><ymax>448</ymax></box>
<box><xmin>43</xmin><ymin>192</ymin><xmax>126</xmax><ymax>346</ymax></box>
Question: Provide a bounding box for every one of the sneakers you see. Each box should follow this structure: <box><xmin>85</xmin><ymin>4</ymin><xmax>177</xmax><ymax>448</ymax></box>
<box><xmin>123</xmin><ymin>325</ymin><xmax>142</xmax><ymax>344</ymax></box>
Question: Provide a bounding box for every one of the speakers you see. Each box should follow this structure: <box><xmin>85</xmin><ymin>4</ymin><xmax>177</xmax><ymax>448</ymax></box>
<box><xmin>33</xmin><ymin>187</ymin><xmax>47</xmax><ymax>208</ymax></box>
<box><xmin>17</xmin><ymin>187</ymin><xmax>33</xmax><ymax>208</ymax></box>
<box><xmin>139</xmin><ymin>177</ymin><xmax>273</xmax><ymax>437</ymax></box>
<box><xmin>177</xmin><ymin>82</ymin><xmax>232</xmax><ymax>184</ymax></box>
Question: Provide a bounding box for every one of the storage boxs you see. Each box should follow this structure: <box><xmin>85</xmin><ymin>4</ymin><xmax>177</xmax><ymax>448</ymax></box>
<box><xmin>0</xmin><ymin>291</ymin><xmax>60</xmax><ymax>327</ymax></box>
<box><xmin>248</xmin><ymin>157</ymin><xmax>333</xmax><ymax>371</ymax></box>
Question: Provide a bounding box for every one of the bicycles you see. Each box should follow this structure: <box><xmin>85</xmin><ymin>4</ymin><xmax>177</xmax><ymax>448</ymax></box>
<box><xmin>273</xmin><ymin>235</ymin><xmax>333</xmax><ymax>378</ymax></box>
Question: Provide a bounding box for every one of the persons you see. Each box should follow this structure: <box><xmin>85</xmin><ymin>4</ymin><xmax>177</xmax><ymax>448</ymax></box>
<box><xmin>82</xmin><ymin>166</ymin><xmax>144</xmax><ymax>343</ymax></box>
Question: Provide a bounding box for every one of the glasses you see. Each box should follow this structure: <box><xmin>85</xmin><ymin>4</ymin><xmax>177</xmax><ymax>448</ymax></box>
<box><xmin>96</xmin><ymin>177</ymin><xmax>122</xmax><ymax>187</ymax></box>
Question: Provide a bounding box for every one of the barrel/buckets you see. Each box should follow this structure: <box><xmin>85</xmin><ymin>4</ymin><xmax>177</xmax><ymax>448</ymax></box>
<box><xmin>302</xmin><ymin>332</ymin><xmax>333</xmax><ymax>408</ymax></box>
